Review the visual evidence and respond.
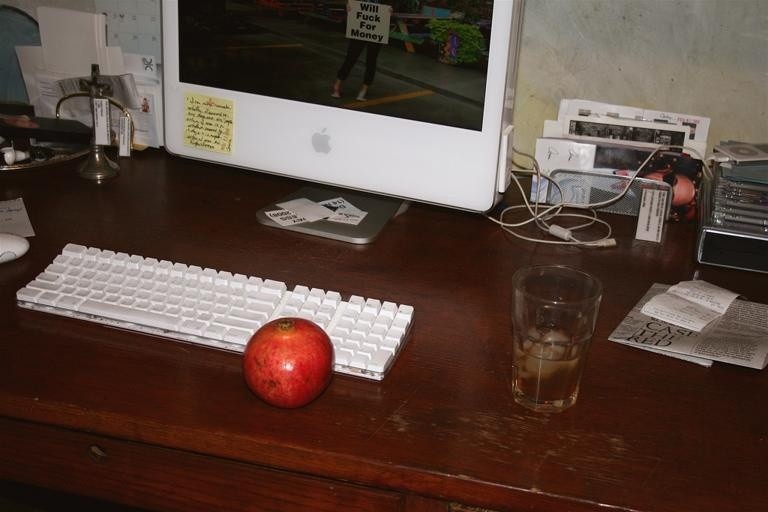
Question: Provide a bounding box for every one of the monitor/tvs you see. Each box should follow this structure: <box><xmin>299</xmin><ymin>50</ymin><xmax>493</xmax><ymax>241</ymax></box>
<box><xmin>159</xmin><ymin>0</ymin><xmax>526</xmax><ymax>244</ymax></box>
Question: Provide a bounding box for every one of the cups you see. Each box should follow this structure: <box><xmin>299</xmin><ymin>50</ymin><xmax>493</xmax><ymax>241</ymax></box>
<box><xmin>510</xmin><ymin>264</ymin><xmax>605</xmax><ymax>417</ymax></box>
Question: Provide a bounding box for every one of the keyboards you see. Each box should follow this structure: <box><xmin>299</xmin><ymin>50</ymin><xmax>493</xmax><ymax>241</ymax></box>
<box><xmin>17</xmin><ymin>244</ymin><xmax>416</xmax><ymax>381</ymax></box>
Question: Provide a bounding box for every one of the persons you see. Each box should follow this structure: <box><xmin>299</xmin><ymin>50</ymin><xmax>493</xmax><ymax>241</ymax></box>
<box><xmin>329</xmin><ymin>0</ymin><xmax>395</xmax><ymax>103</ymax></box>
<box><xmin>590</xmin><ymin>165</ymin><xmax>697</xmax><ymax>215</ymax></box>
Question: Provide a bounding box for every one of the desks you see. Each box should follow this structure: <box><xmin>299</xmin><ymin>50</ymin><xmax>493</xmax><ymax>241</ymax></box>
<box><xmin>0</xmin><ymin>100</ymin><xmax>768</xmax><ymax>512</ymax></box>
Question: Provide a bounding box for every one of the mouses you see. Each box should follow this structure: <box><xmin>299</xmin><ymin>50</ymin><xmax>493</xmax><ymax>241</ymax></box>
<box><xmin>0</xmin><ymin>233</ymin><xmax>30</xmax><ymax>263</ymax></box>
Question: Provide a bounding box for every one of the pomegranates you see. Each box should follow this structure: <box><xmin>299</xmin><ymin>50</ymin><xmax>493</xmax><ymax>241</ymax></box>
<box><xmin>242</xmin><ymin>317</ymin><xmax>335</xmax><ymax>409</ymax></box>
